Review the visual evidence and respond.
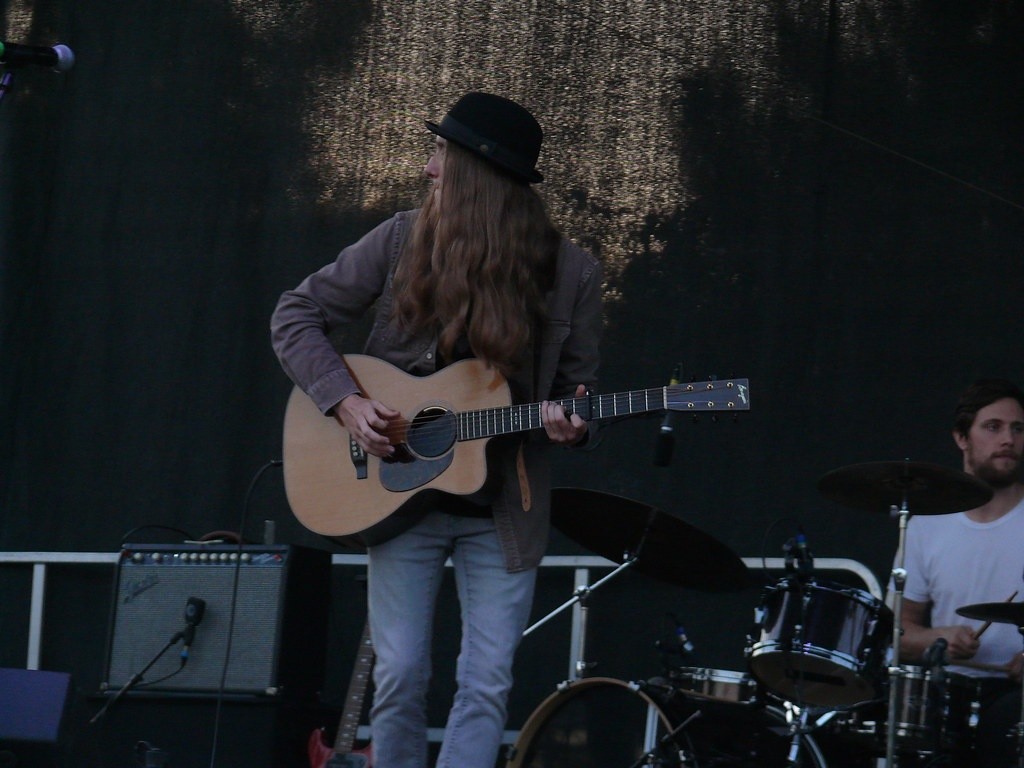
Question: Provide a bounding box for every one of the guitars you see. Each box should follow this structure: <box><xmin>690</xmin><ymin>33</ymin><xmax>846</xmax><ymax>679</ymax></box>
<box><xmin>306</xmin><ymin>606</ymin><xmax>379</xmax><ymax>767</ymax></box>
<box><xmin>283</xmin><ymin>354</ymin><xmax>750</xmax><ymax>543</ymax></box>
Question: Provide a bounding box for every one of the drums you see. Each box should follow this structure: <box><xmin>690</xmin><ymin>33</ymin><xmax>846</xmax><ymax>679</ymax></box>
<box><xmin>500</xmin><ymin>677</ymin><xmax>830</xmax><ymax>768</ymax></box>
<box><xmin>672</xmin><ymin>666</ymin><xmax>752</xmax><ymax>707</ymax></box>
<box><xmin>833</xmin><ymin>663</ymin><xmax>952</xmax><ymax>747</ymax></box>
<box><xmin>741</xmin><ymin>573</ymin><xmax>893</xmax><ymax>710</ymax></box>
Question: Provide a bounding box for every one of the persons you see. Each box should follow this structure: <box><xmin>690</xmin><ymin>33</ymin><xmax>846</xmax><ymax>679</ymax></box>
<box><xmin>271</xmin><ymin>93</ymin><xmax>603</xmax><ymax>768</ymax></box>
<box><xmin>884</xmin><ymin>382</ymin><xmax>1024</xmax><ymax>768</ymax></box>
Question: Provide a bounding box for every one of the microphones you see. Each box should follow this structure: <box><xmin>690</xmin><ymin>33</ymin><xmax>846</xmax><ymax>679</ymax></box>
<box><xmin>181</xmin><ymin>596</ymin><xmax>206</xmax><ymax>666</ymax></box>
<box><xmin>0</xmin><ymin>40</ymin><xmax>75</xmax><ymax>72</ymax></box>
<box><xmin>798</xmin><ymin>525</ymin><xmax>817</xmax><ymax>594</ymax></box>
<box><xmin>928</xmin><ymin>638</ymin><xmax>947</xmax><ymax>667</ymax></box>
<box><xmin>672</xmin><ymin>615</ymin><xmax>699</xmax><ymax>666</ymax></box>
<box><xmin>660</xmin><ymin>367</ymin><xmax>679</xmax><ymax>438</ymax></box>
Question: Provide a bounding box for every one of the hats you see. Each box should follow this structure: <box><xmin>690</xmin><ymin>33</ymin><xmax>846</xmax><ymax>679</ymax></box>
<box><xmin>424</xmin><ymin>91</ymin><xmax>544</xmax><ymax>184</ymax></box>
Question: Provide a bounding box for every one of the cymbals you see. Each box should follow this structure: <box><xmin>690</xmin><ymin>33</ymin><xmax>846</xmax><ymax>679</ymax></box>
<box><xmin>811</xmin><ymin>458</ymin><xmax>987</xmax><ymax>521</ymax></box>
<box><xmin>547</xmin><ymin>484</ymin><xmax>736</xmax><ymax>580</ymax></box>
<box><xmin>954</xmin><ymin>602</ymin><xmax>1024</xmax><ymax>626</ymax></box>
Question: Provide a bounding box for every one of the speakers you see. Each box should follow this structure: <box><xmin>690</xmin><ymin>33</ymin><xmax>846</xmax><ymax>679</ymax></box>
<box><xmin>0</xmin><ymin>542</ymin><xmax>344</xmax><ymax>768</ymax></box>
